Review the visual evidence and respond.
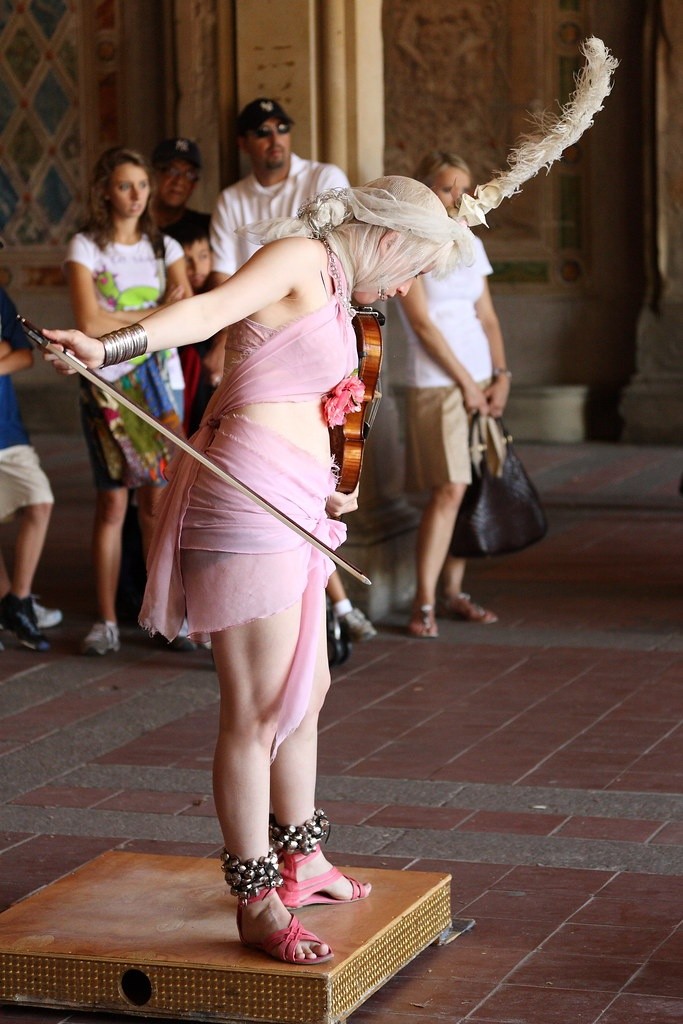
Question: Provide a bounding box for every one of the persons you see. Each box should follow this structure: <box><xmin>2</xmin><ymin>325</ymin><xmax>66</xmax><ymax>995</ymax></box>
<box><xmin>0</xmin><ymin>282</ymin><xmax>63</xmax><ymax>656</ymax></box>
<box><xmin>69</xmin><ymin>134</ymin><xmax>193</xmax><ymax>656</ymax></box>
<box><xmin>139</xmin><ymin>137</ymin><xmax>212</xmax><ymax>441</ymax></box>
<box><xmin>43</xmin><ymin>175</ymin><xmax>473</xmax><ymax>965</ymax></box>
<box><xmin>397</xmin><ymin>145</ymin><xmax>509</xmax><ymax>646</ymax></box>
<box><xmin>211</xmin><ymin>95</ymin><xmax>347</xmax><ymax>375</ymax></box>
<box><xmin>326</xmin><ymin>567</ymin><xmax>376</xmax><ymax>663</ymax></box>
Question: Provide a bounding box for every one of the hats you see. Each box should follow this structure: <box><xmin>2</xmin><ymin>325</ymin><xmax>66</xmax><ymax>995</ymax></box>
<box><xmin>152</xmin><ymin>138</ymin><xmax>203</xmax><ymax>170</ymax></box>
<box><xmin>238</xmin><ymin>98</ymin><xmax>294</xmax><ymax>137</ymax></box>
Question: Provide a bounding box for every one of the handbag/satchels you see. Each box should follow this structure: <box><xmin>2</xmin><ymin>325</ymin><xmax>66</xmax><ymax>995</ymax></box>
<box><xmin>450</xmin><ymin>411</ymin><xmax>548</xmax><ymax>557</ymax></box>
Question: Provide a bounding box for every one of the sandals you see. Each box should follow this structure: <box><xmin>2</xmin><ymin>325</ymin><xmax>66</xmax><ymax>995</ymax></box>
<box><xmin>275</xmin><ymin>844</ymin><xmax>370</xmax><ymax>909</ymax></box>
<box><xmin>237</xmin><ymin>889</ymin><xmax>334</xmax><ymax>965</ymax></box>
<box><xmin>436</xmin><ymin>593</ymin><xmax>498</xmax><ymax>625</ymax></box>
<box><xmin>408</xmin><ymin>604</ymin><xmax>439</xmax><ymax>638</ymax></box>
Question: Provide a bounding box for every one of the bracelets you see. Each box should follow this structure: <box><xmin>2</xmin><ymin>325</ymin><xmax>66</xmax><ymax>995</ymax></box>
<box><xmin>493</xmin><ymin>367</ymin><xmax>514</xmax><ymax>384</ymax></box>
<box><xmin>96</xmin><ymin>325</ymin><xmax>151</xmax><ymax>370</ymax></box>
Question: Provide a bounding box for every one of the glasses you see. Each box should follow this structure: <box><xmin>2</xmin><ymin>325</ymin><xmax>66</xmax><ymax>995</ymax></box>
<box><xmin>161</xmin><ymin>168</ymin><xmax>199</xmax><ymax>182</ymax></box>
<box><xmin>255</xmin><ymin>123</ymin><xmax>290</xmax><ymax>138</ymax></box>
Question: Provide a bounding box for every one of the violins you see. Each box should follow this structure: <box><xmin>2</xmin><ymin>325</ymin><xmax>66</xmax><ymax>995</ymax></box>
<box><xmin>329</xmin><ymin>304</ymin><xmax>386</xmax><ymax>494</ymax></box>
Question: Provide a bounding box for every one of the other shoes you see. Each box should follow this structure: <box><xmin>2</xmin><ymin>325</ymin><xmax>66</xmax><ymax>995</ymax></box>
<box><xmin>31</xmin><ymin>595</ymin><xmax>62</xmax><ymax>628</ymax></box>
<box><xmin>81</xmin><ymin>621</ymin><xmax>119</xmax><ymax>656</ymax></box>
<box><xmin>1</xmin><ymin>593</ymin><xmax>51</xmax><ymax>651</ymax></box>
<box><xmin>166</xmin><ymin>619</ymin><xmax>197</xmax><ymax>651</ymax></box>
<box><xmin>339</xmin><ymin>607</ymin><xmax>377</xmax><ymax>643</ymax></box>
